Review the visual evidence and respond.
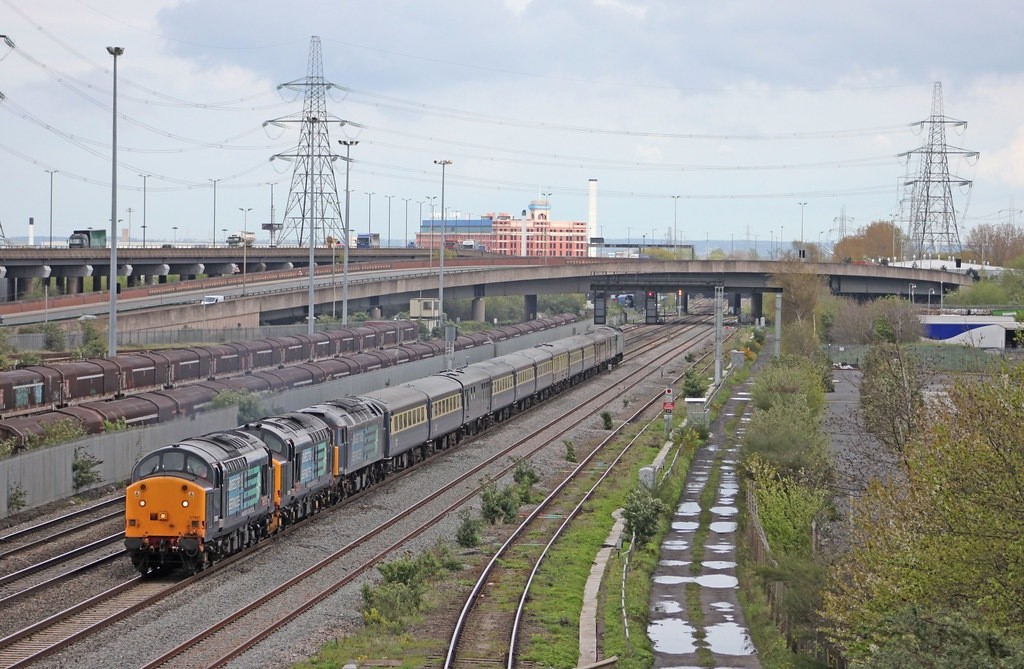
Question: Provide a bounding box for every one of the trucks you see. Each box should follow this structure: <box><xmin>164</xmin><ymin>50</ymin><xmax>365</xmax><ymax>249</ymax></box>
<box><xmin>200</xmin><ymin>295</ymin><xmax>224</xmax><ymax>304</ymax></box>
<box><xmin>67</xmin><ymin>230</ymin><xmax>106</xmax><ymax>249</ymax></box>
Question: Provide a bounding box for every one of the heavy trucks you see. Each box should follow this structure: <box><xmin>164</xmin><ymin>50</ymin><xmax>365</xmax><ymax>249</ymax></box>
<box><xmin>443</xmin><ymin>239</ymin><xmax>463</xmax><ymax>250</ymax></box>
<box><xmin>355</xmin><ymin>233</ymin><xmax>380</xmax><ymax>248</ymax></box>
<box><xmin>463</xmin><ymin>239</ymin><xmax>479</xmax><ymax>251</ymax></box>
<box><xmin>228</xmin><ymin>231</ymin><xmax>255</xmax><ymax>247</ymax></box>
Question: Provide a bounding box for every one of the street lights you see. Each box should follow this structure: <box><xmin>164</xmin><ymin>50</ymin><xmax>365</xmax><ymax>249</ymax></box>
<box><xmin>106</xmin><ymin>45</ymin><xmax>123</xmax><ymax>358</ymax></box>
<box><xmin>139</xmin><ymin>174</ymin><xmax>151</xmax><ymax>249</ymax></box>
<box><xmin>208</xmin><ymin>179</ymin><xmax>278</xmax><ymax>245</ymax></box>
<box><xmin>338</xmin><ymin>140</ymin><xmax>359</xmax><ymax>326</ymax></box>
<box><xmin>44</xmin><ymin>169</ymin><xmax>59</xmax><ymax>247</ymax></box>
<box><xmin>435</xmin><ymin>160</ymin><xmax>452</xmax><ymax>311</ymax></box>
<box><xmin>344</xmin><ymin>189</ymin><xmax>471</xmax><ymax>248</ymax></box>
<box><xmin>543</xmin><ymin>193</ymin><xmax>899</xmax><ymax>261</ymax></box>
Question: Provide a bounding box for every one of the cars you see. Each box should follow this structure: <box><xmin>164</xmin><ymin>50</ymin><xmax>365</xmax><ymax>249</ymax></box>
<box><xmin>162</xmin><ymin>244</ymin><xmax>176</xmax><ymax>248</ymax></box>
<box><xmin>78</xmin><ymin>315</ymin><xmax>97</xmax><ymax>320</ymax></box>
<box><xmin>608</xmin><ymin>252</ymin><xmax>649</xmax><ymax>259</ymax></box>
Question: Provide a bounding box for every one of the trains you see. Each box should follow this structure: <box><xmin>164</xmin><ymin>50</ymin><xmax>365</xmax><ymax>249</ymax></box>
<box><xmin>123</xmin><ymin>325</ymin><xmax>624</xmax><ymax>577</ymax></box>
<box><xmin>0</xmin><ymin>312</ymin><xmax>579</xmax><ymax>458</ymax></box>
<box><xmin>0</xmin><ymin>321</ymin><xmax>420</xmax><ymax>417</ymax></box>
